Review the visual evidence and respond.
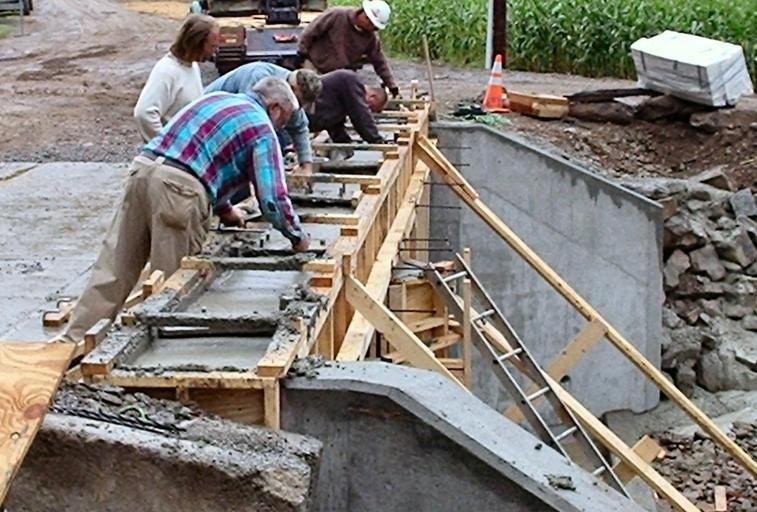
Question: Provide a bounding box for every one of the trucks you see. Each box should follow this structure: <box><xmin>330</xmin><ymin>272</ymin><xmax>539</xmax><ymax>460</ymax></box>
<box><xmin>197</xmin><ymin>0</ymin><xmax>329</xmax><ymax>74</ymax></box>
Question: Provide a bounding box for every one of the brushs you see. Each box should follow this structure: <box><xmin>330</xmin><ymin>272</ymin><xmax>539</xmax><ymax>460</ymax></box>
<box><xmin>233</xmin><ymin>203</ymin><xmax>263</xmax><ymax>220</ymax></box>
<box><xmin>210</xmin><ymin>219</ymin><xmax>265</xmax><ymax>232</ymax></box>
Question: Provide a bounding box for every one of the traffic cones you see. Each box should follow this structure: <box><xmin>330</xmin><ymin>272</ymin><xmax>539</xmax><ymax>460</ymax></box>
<box><xmin>480</xmin><ymin>54</ymin><xmax>512</xmax><ymax>113</ymax></box>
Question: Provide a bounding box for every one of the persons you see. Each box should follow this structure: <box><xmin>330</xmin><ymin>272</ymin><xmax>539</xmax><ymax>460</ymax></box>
<box><xmin>42</xmin><ymin>76</ymin><xmax>311</xmax><ymax>353</ymax></box>
<box><xmin>202</xmin><ymin>59</ymin><xmax>323</xmax><ymax>176</ymax></box>
<box><xmin>304</xmin><ymin>68</ymin><xmax>387</xmax><ymax>147</ymax></box>
<box><xmin>130</xmin><ymin>13</ymin><xmax>221</xmax><ymax>144</ymax></box>
<box><xmin>292</xmin><ymin>0</ymin><xmax>401</xmax><ymax>96</ymax></box>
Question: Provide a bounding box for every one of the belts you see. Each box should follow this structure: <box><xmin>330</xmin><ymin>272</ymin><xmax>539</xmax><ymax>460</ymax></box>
<box><xmin>139</xmin><ymin>149</ymin><xmax>211</xmax><ymax>194</ymax></box>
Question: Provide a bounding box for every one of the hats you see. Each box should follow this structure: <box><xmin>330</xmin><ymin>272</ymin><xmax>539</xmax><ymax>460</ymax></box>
<box><xmin>288</xmin><ymin>68</ymin><xmax>322</xmax><ymax>115</ymax></box>
<box><xmin>362</xmin><ymin>0</ymin><xmax>392</xmax><ymax>30</ymax></box>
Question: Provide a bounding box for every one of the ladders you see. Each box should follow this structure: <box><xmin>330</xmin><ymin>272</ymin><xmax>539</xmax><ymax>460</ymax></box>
<box><xmin>422</xmin><ymin>253</ymin><xmax>634</xmax><ymax>504</ymax></box>
